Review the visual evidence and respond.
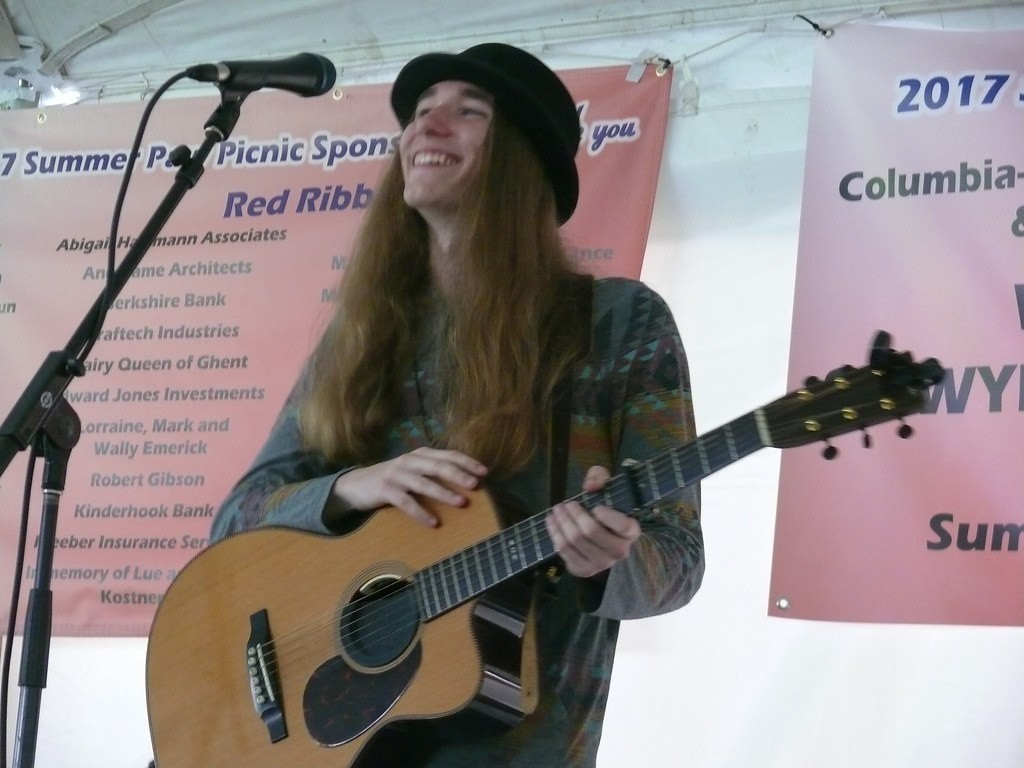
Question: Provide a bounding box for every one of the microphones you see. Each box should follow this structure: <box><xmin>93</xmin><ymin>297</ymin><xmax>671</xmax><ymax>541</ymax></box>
<box><xmin>185</xmin><ymin>53</ymin><xmax>336</xmax><ymax>97</ymax></box>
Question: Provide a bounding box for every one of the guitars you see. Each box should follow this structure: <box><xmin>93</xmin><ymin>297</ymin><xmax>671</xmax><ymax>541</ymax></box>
<box><xmin>142</xmin><ymin>328</ymin><xmax>946</xmax><ymax>768</ymax></box>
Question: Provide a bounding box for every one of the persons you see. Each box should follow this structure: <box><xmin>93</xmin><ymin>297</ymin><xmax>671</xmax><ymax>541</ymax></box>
<box><xmin>213</xmin><ymin>42</ymin><xmax>705</xmax><ymax>767</ymax></box>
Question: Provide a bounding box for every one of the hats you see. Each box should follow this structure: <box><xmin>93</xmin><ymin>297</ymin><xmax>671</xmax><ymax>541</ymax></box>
<box><xmin>390</xmin><ymin>43</ymin><xmax>581</xmax><ymax>228</ymax></box>
